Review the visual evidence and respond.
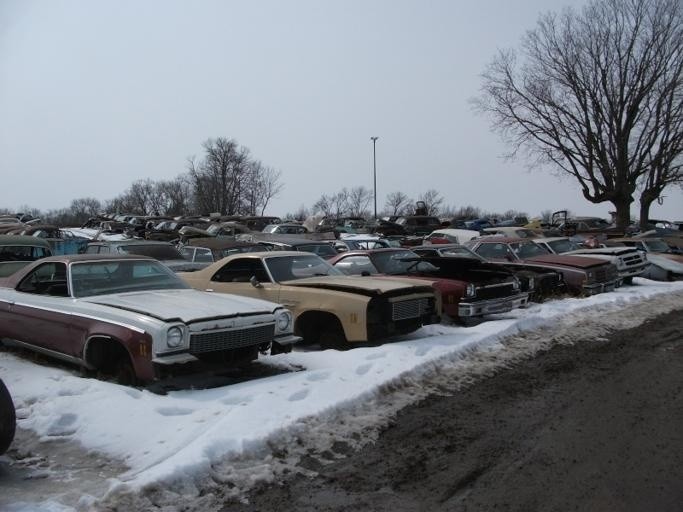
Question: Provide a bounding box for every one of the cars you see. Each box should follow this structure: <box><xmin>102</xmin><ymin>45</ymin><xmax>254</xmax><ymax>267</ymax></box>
<box><xmin>239</xmin><ymin>215</ymin><xmax>281</xmax><ymax>231</ymax></box>
<box><xmin>322</xmin><ymin>239</ymin><xmax>390</xmax><ymax>250</ymax></box>
<box><xmin>534</xmin><ymin>236</ymin><xmax>652</xmax><ymax>277</ymax></box>
<box><xmin>179</xmin><ymin>240</ymin><xmax>269</xmax><ymax>262</ymax></box>
<box><xmin>212</xmin><ymin>215</ymin><xmax>240</xmax><ymax>222</ymax></box>
<box><xmin>0</xmin><ymin>234</ymin><xmax>55</xmax><ymax>279</ymax></box>
<box><xmin>178</xmin><ymin>221</ymin><xmax>259</xmax><ymax>256</ymax></box>
<box><xmin>0</xmin><ymin>252</ymin><xmax>304</xmax><ymax>389</ymax></box>
<box><xmin>606</xmin><ymin>236</ymin><xmax>683</xmax><ymax>278</ymax></box>
<box><xmin>129</xmin><ymin>216</ymin><xmax>149</xmax><ymax>229</ymax></box>
<box><xmin>234</xmin><ymin>214</ymin><xmax>327</xmax><ymax>242</ymax></box>
<box><xmin>396</xmin><ymin>215</ymin><xmax>441</xmax><ymax>229</ymax></box>
<box><xmin>257</xmin><ymin>238</ymin><xmax>339</xmax><ymax>260</ymax></box>
<box><xmin>145</xmin><ymin>220</ymin><xmax>208</xmax><ymax>241</ymax></box>
<box><xmin>327</xmin><ymin>247</ymin><xmax>528</xmax><ymax>317</ymax></box>
<box><xmin>465</xmin><ymin>238</ymin><xmax>618</xmax><ymax>296</ymax></box>
<box><xmin>175</xmin><ymin>251</ymin><xmax>443</xmax><ymax>344</ymax></box>
<box><xmin>399</xmin><ymin>227</ymin><xmax>480</xmax><ymax>248</ymax></box>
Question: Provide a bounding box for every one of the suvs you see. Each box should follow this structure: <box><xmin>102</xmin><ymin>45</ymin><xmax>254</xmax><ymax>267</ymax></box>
<box><xmin>84</xmin><ymin>240</ymin><xmax>208</xmax><ymax>274</ymax></box>
<box><xmin>20</xmin><ymin>225</ymin><xmax>60</xmax><ymax>238</ymax></box>
<box><xmin>483</xmin><ymin>226</ymin><xmax>539</xmax><ymax>237</ymax></box>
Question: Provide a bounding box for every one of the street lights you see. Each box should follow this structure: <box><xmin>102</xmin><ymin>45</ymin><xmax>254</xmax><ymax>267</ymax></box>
<box><xmin>371</xmin><ymin>136</ymin><xmax>378</xmax><ymax>219</ymax></box>
